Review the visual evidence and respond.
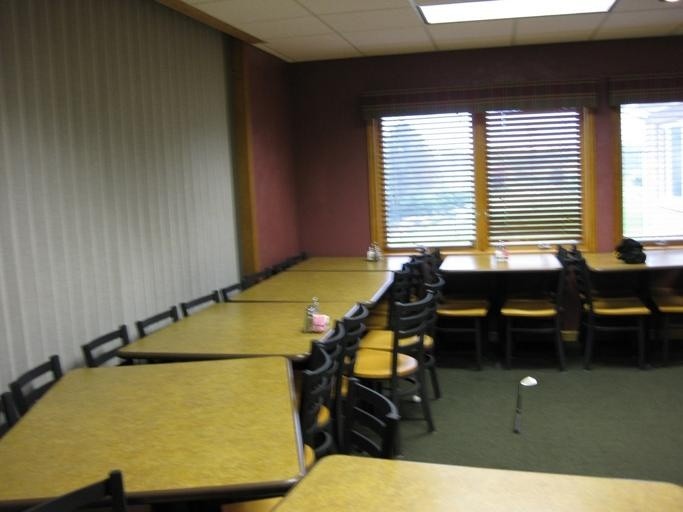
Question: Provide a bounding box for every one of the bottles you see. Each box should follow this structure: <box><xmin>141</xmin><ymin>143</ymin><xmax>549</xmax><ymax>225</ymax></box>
<box><xmin>366</xmin><ymin>244</ymin><xmax>376</xmax><ymax>261</ymax></box>
<box><xmin>303</xmin><ymin>296</ymin><xmax>321</xmax><ymax>333</ymax></box>
<box><xmin>495</xmin><ymin>245</ymin><xmax>505</xmax><ymax>261</ymax></box>
